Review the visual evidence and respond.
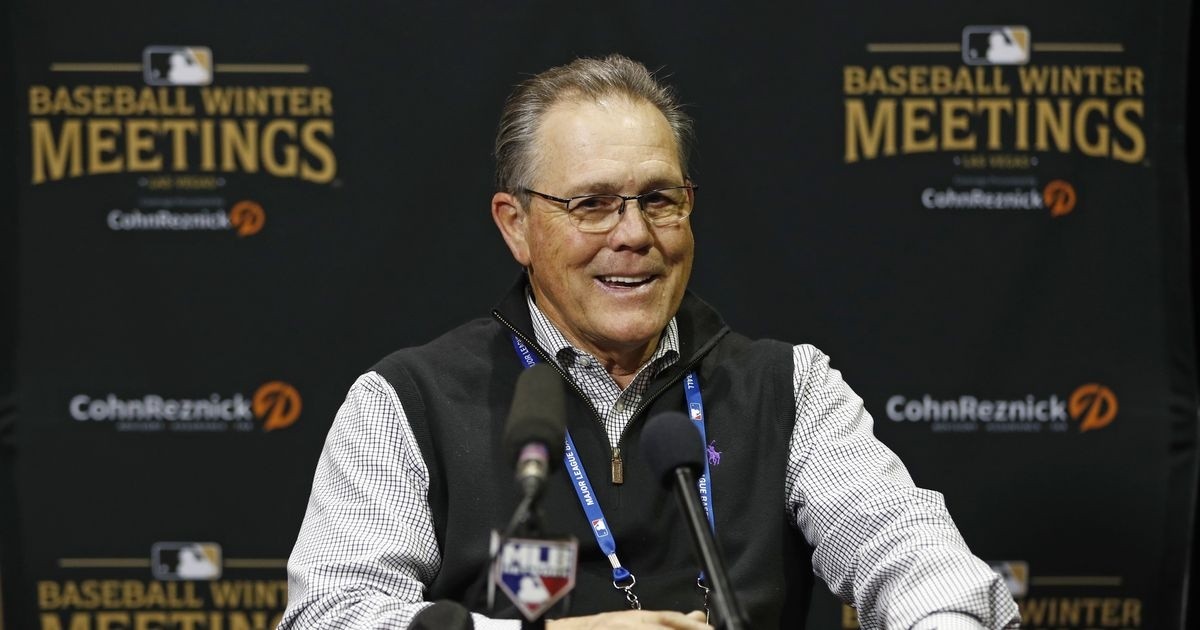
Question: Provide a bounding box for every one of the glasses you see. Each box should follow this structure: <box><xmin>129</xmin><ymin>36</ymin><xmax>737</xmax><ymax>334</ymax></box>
<box><xmin>514</xmin><ymin>175</ymin><xmax>699</xmax><ymax>234</ymax></box>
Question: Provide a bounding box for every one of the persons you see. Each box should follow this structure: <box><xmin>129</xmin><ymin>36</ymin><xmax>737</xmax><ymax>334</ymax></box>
<box><xmin>278</xmin><ymin>56</ymin><xmax>1021</xmax><ymax>630</ymax></box>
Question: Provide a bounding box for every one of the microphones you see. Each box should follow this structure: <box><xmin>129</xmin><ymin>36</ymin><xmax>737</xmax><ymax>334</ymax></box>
<box><xmin>503</xmin><ymin>364</ymin><xmax>566</xmax><ymax>489</ymax></box>
<box><xmin>638</xmin><ymin>412</ymin><xmax>752</xmax><ymax>630</ymax></box>
